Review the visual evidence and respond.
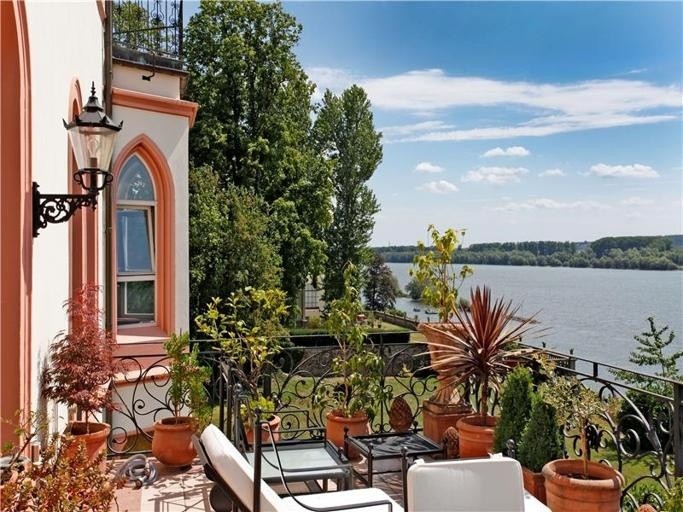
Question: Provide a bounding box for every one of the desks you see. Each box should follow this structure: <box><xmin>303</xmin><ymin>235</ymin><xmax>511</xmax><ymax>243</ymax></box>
<box><xmin>339</xmin><ymin>421</ymin><xmax>448</xmax><ymax>495</ymax></box>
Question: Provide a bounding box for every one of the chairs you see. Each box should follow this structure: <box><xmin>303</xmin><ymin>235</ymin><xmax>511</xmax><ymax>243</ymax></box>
<box><xmin>190</xmin><ymin>356</ymin><xmax>404</xmax><ymax>512</ymax></box>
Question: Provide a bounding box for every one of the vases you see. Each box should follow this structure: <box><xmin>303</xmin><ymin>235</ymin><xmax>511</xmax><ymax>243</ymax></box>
<box><xmin>0</xmin><ymin>455</ymin><xmax>32</xmax><ymax>509</ymax></box>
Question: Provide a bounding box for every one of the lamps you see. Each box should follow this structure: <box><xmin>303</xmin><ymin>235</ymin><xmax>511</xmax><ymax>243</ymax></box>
<box><xmin>26</xmin><ymin>80</ymin><xmax>124</xmax><ymax>237</ymax></box>
